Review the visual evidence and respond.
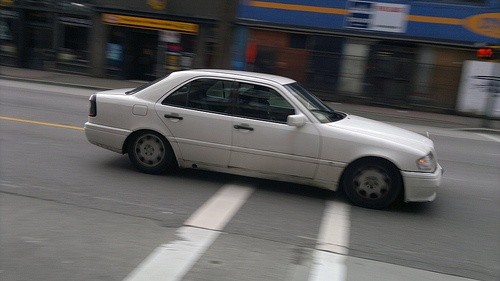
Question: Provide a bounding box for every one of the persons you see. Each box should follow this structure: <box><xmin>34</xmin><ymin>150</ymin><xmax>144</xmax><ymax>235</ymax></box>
<box><xmin>242</xmin><ymin>85</ymin><xmax>276</xmax><ymax>120</ymax></box>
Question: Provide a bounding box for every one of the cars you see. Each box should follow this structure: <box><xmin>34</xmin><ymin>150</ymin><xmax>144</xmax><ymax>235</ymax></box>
<box><xmin>84</xmin><ymin>67</ymin><xmax>443</xmax><ymax>209</ymax></box>
